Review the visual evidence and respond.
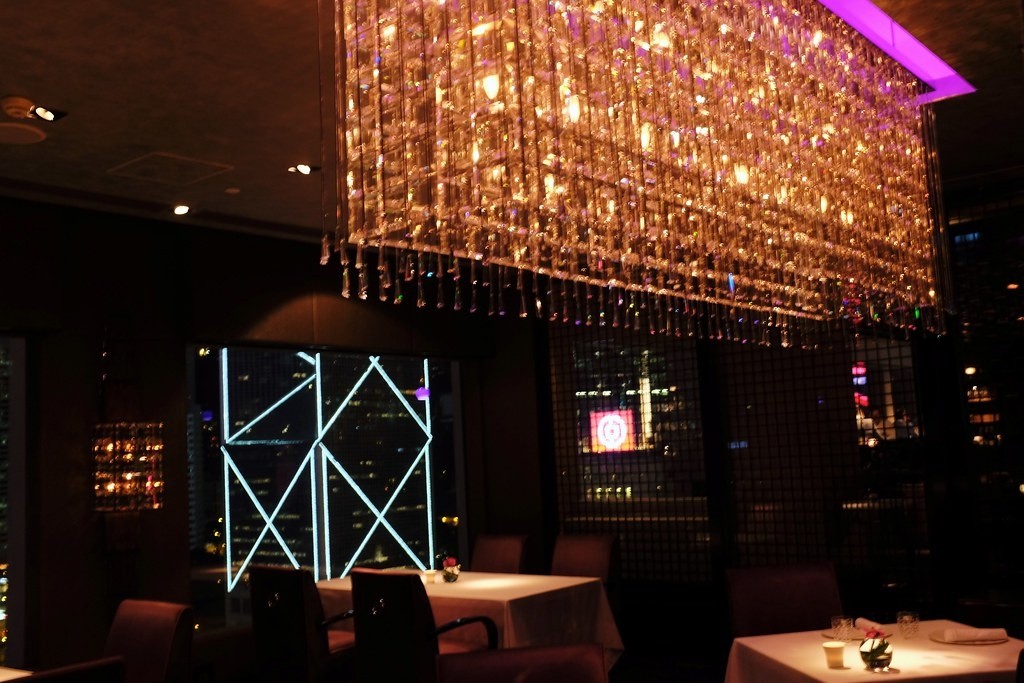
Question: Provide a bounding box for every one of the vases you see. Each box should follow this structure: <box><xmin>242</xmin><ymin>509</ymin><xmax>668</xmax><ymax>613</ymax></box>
<box><xmin>859</xmin><ymin>638</ymin><xmax>893</xmax><ymax>672</ymax></box>
<box><xmin>441</xmin><ymin>569</ymin><xmax>458</xmax><ymax>583</ymax></box>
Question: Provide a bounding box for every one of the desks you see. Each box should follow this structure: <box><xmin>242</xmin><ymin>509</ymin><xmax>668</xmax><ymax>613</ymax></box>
<box><xmin>318</xmin><ymin>569</ymin><xmax>625</xmax><ymax>683</ymax></box>
<box><xmin>723</xmin><ymin>619</ymin><xmax>1024</xmax><ymax>683</ymax></box>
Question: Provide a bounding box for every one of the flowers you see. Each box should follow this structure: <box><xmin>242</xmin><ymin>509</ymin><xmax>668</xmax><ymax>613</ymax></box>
<box><xmin>865</xmin><ymin>627</ymin><xmax>893</xmax><ymax>639</ymax></box>
<box><xmin>443</xmin><ymin>557</ymin><xmax>461</xmax><ymax>575</ymax></box>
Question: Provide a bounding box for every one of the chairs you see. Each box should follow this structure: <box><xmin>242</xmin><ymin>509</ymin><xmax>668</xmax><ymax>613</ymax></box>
<box><xmin>247</xmin><ymin>563</ymin><xmax>356</xmax><ymax>683</ymax></box>
<box><xmin>549</xmin><ymin>532</ymin><xmax>622</xmax><ymax>609</ymax></box>
<box><xmin>0</xmin><ymin>655</ymin><xmax>127</xmax><ymax>683</ymax></box>
<box><xmin>101</xmin><ymin>598</ymin><xmax>194</xmax><ymax>683</ymax></box>
<box><xmin>470</xmin><ymin>533</ymin><xmax>537</xmax><ymax>573</ymax></box>
<box><xmin>351</xmin><ymin>567</ymin><xmax>497</xmax><ymax>683</ymax></box>
<box><xmin>727</xmin><ymin>560</ymin><xmax>844</xmax><ymax>637</ymax></box>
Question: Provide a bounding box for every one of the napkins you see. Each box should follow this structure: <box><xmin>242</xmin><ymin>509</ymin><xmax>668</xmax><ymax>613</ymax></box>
<box><xmin>855</xmin><ymin>618</ymin><xmax>882</xmax><ymax>630</ymax></box>
<box><xmin>944</xmin><ymin>627</ymin><xmax>1008</xmax><ymax>643</ymax></box>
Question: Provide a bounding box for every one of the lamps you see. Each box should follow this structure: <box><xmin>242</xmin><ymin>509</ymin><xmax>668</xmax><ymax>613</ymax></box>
<box><xmin>314</xmin><ymin>0</ymin><xmax>950</xmax><ymax>352</ymax></box>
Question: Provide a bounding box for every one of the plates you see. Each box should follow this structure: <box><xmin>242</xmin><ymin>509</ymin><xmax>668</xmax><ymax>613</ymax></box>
<box><xmin>928</xmin><ymin>630</ymin><xmax>1008</xmax><ymax>645</ymax></box>
<box><xmin>822</xmin><ymin>628</ymin><xmax>893</xmax><ymax>639</ymax></box>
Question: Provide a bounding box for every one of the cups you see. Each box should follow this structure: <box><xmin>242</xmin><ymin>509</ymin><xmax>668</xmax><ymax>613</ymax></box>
<box><xmin>423</xmin><ymin>570</ymin><xmax>437</xmax><ymax>584</ymax></box>
<box><xmin>823</xmin><ymin>642</ymin><xmax>846</xmax><ymax>666</ymax></box>
<box><xmin>897</xmin><ymin>612</ymin><xmax>919</xmax><ymax>639</ymax></box>
<box><xmin>831</xmin><ymin>617</ymin><xmax>853</xmax><ymax>643</ymax></box>
